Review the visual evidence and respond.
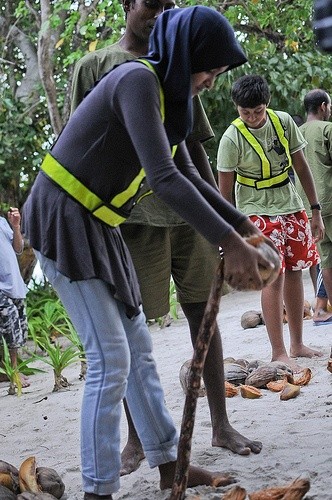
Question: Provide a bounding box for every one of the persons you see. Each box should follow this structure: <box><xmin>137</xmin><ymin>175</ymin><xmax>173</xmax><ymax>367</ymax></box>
<box><xmin>69</xmin><ymin>0</ymin><xmax>263</xmax><ymax>478</ymax></box>
<box><xmin>21</xmin><ymin>7</ymin><xmax>284</xmax><ymax>500</ymax></box>
<box><xmin>313</xmin><ymin>263</ymin><xmax>332</xmax><ymax>326</ymax></box>
<box><xmin>217</xmin><ymin>76</ymin><xmax>323</xmax><ymax>373</ymax></box>
<box><xmin>0</xmin><ymin>207</ymin><xmax>30</xmax><ymax>388</ymax></box>
<box><xmin>290</xmin><ymin>89</ymin><xmax>332</xmax><ymax>307</ymax></box>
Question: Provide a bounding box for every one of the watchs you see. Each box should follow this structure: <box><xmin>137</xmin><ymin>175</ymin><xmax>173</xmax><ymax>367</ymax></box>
<box><xmin>310</xmin><ymin>203</ymin><xmax>320</xmax><ymax>210</ymax></box>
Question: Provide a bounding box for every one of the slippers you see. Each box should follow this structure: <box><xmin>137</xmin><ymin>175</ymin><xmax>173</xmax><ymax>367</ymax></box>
<box><xmin>313</xmin><ymin>315</ymin><xmax>332</xmax><ymax>326</ymax></box>
